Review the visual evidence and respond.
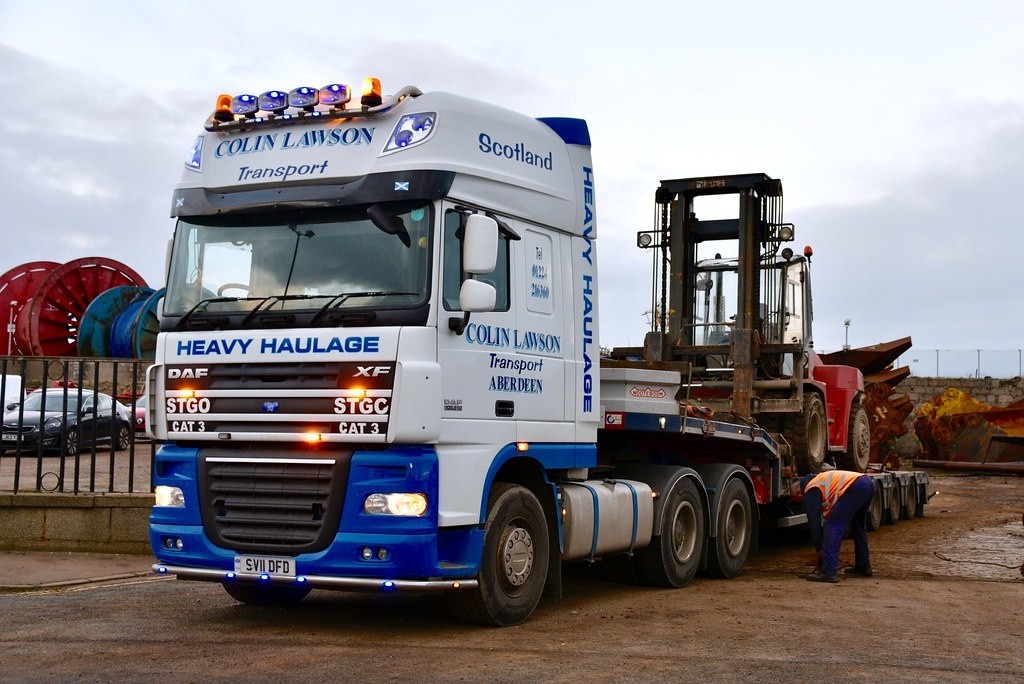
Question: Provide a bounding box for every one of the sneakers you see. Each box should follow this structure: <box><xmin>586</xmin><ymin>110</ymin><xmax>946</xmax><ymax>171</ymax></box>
<box><xmin>806</xmin><ymin>567</ymin><xmax>836</xmax><ymax>582</ymax></box>
<box><xmin>845</xmin><ymin>567</ymin><xmax>873</xmax><ymax>576</ymax></box>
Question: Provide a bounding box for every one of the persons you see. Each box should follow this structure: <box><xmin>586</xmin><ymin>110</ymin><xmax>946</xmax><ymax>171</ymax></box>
<box><xmin>791</xmin><ymin>470</ymin><xmax>876</xmax><ymax>583</ymax></box>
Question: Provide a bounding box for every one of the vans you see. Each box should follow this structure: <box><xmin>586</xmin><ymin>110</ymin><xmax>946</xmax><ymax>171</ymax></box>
<box><xmin>0</xmin><ymin>375</ymin><xmax>28</xmax><ymax>417</ymax></box>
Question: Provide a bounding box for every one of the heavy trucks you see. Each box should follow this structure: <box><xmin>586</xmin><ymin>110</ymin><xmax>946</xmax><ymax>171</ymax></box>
<box><xmin>143</xmin><ymin>77</ymin><xmax>932</xmax><ymax>630</ymax></box>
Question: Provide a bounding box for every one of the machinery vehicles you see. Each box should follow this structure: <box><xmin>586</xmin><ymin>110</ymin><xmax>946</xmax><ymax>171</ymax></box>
<box><xmin>606</xmin><ymin>173</ymin><xmax>913</xmax><ymax>477</ymax></box>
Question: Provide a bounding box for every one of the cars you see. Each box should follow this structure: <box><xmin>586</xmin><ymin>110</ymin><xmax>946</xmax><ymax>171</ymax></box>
<box><xmin>1</xmin><ymin>387</ymin><xmax>132</xmax><ymax>456</ymax></box>
<box><xmin>125</xmin><ymin>395</ymin><xmax>146</xmax><ymax>432</ymax></box>
